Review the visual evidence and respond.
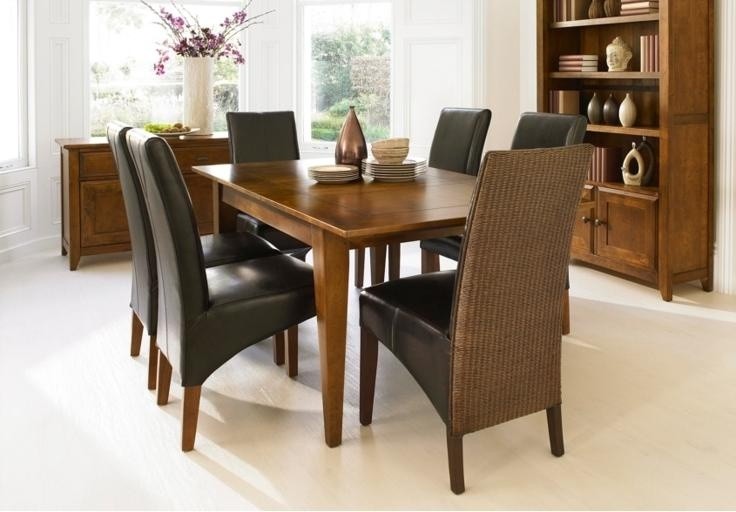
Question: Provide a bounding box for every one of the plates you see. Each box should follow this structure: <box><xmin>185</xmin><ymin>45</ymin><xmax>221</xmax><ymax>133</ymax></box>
<box><xmin>308</xmin><ymin>165</ymin><xmax>361</xmax><ymax>183</ymax></box>
<box><xmin>157</xmin><ymin>128</ymin><xmax>201</xmax><ymax>135</ymax></box>
<box><xmin>361</xmin><ymin>160</ymin><xmax>428</xmax><ymax>182</ymax></box>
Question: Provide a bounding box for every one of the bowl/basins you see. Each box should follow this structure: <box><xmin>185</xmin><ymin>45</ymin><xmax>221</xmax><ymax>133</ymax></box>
<box><xmin>371</xmin><ymin>138</ymin><xmax>410</xmax><ymax>163</ymax></box>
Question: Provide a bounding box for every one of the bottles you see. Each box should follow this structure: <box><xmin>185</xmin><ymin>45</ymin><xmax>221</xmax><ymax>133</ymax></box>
<box><xmin>588</xmin><ymin>0</ymin><xmax>621</xmax><ymax>20</ymax></box>
<box><xmin>335</xmin><ymin>107</ymin><xmax>367</xmax><ymax>182</ymax></box>
<box><xmin>585</xmin><ymin>90</ymin><xmax>638</xmax><ymax>127</ymax></box>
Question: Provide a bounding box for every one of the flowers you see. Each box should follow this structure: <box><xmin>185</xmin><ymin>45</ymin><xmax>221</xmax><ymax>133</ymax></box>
<box><xmin>139</xmin><ymin>1</ymin><xmax>277</xmax><ymax>76</ymax></box>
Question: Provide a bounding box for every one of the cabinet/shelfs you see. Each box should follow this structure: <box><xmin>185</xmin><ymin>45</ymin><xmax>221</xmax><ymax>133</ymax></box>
<box><xmin>535</xmin><ymin>0</ymin><xmax>716</xmax><ymax>302</ymax></box>
<box><xmin>55</xmin><ymin>134</ymin><xmax>233</xmax><ymax>271</ymax></box>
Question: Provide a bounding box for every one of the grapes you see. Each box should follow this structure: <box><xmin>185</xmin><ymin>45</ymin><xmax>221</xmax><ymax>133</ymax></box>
<box><xmin>144</xmin><ymin>123</ymin><xmax>170</xmax><ymax>133</ymax></box>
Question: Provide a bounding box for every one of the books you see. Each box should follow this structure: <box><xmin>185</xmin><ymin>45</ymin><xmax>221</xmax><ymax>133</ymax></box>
<box><xmin>552</xmin><ymin>0</ymin><xmax>586</xmax><ymax>22</ymax></box>
<box><xmin>587</xmin><ymin>145</ymin><xmax>618</xmax><ymax>183</ymax></box>
<box><xmin>559</xmin><ymin>55</ymin><xmax>599</xmax><ymax>73</ymax></box>
<box><xmin>620</xmin><ymin>0</ymin><xmax>659</xmax><ymax>15</ymax></box>
<box><xmin>639</xmin><ymin>35</ymin><xmax>659</xmax><ymax>73</ymax></box>
<box><xmin>549</xmin><ymin>90</ymin><xmax>580</xmax><ymax>115</ymax></box>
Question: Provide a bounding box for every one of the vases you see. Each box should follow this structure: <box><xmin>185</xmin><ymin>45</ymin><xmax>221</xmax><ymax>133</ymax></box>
<box><xmin>180</xmin><ymin>55</ymin><xmax>214</xmax><ymax>134</ymax></box>
<box><xmin>334</xmin><ymin>105</ymin><xmax>367</xmax><ymax>167</ymax></box>
<box><xmin>586</xmin><ymin>90</ymin><xmax>637</xmax><ymax>130</ymax></box>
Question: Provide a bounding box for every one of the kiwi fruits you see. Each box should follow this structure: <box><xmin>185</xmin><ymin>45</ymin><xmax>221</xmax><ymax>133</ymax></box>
<box><xmin>162</xmin><ymin>123</ymin><xmax>190</xmax><ymax>133</ymax></box>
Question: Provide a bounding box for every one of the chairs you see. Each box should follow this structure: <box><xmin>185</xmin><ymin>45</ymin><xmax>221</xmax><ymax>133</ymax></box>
<box><xmin>127</xmin><ymin>127</ymin><xmax>317</xmax><ymax>453</ymax></box>
<box><xmin>226</xmin><ymin>110</ymin><xmax>347</xmax><ymax>378</ymax></box>
<box><xmin>354</xmin><ymin>107</ymin><xmax>491</xmax><ymax>289</ymax></box>
<box><xmin>419</xmin><ymin>112</ymin><xmax>587</xmax><ymax>336</ymax></box>
<box><xmin>106</xmin><ymin>119</ymin><xmax>286</xmax><ymax>391</ymax></box>
<box><xmin>359</xmin><ymin>143</ymin><xmax>594</xmax><ymax>495</ymax></box>
